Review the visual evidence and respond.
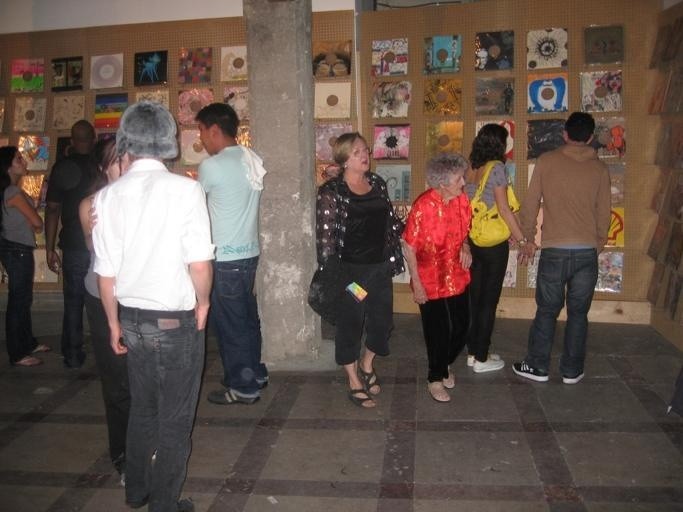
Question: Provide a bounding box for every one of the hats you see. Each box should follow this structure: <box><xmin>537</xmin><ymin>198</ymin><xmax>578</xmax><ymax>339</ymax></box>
<box><xmin>115</xmin><ymin>101</ymin><xmax>180</xmax><ymax>160</ymax></box>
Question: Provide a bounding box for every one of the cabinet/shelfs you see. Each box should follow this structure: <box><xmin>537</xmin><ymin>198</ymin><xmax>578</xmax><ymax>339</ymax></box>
<box><xmin>643</xmin><ymin>0</ymin><xmax>683</xmax><ymax>356</ymax></box>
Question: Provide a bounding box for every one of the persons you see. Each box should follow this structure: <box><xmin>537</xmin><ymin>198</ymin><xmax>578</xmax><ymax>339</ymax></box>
<box><xmin>90</xmin><ymin>98</ymin><xmax>219</xmax><ymax>511</ymax></box>
<box><xmin>402</xmin><ymin>151</ymin><xmax>475</xmax><ymax>404</ymax></box>
<box><xmin>44</xmin><ymin>119</ymin><xmax>107</xmax><ymax>368</ymax></box>
<box><xmin>315</xmin><ymin>131</ymin><xmax>407</xmax><ymax>409</ymax></box>
<box><xmin>463</xmin><ymin>122</ymin><xmax>539</xmax><ymax>374</ymax></box>
<box><xmin>0</xmin><ymin>144</ymin><xmax>54</xmax><ymax>367</ymax></box>
<box><xmin>78</xmin><ymin>134</ymin><xmax>132</xmax><ymax>487</ymax></box>
<box><xmin>194</xmin><ymin>101</ymin><xmax>270</xmax><ymax>404</ymax></box>
<box><xmin>511</xmin><ymin>109</ymin><xmax>612</xmax><ymax>386</ymax></box>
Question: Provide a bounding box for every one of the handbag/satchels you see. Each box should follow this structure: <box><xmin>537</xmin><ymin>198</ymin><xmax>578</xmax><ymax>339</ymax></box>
<box><xmin>307</xmin><ymin>251</ymin><xmax>347</xmax><ymax>326</ymax></box>
<box><xmin>468</xmin><ymin>185</ymin><xmax>520</xmax><ymax>247</ymax></box>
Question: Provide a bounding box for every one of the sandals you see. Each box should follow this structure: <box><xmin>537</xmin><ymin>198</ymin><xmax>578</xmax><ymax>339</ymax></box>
<box><xmin>347</xmin><ymin>386</ymin><xmax>375</xmax><ymax>409</ymax></box>
<box><xmin>358</xmin><ymin>366</ymin><xmax>381</xmax><ymax>395</ymax></box>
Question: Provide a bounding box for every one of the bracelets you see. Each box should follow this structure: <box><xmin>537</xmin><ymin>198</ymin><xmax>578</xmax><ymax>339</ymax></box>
<box><xmin>516</xmin><ymin>237</ymin><xmax>528</xmax><ymax>246</ymax></box>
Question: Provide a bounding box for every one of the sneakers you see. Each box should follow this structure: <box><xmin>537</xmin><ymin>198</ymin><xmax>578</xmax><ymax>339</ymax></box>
<box><xmin>14</xmin><ymin>356</ymin><xmax>42</xmax><ymax>366</ymax></box>
<box><xmin>206</xmin><ymin>386</ymin><xmax>261</xmax><ymax>405</ymax></box>
<box><xmin>429</xmin><ymin>354</ymin><xmax>585</xmax><ymax>402</ymax></box>
<box><xmin>177</xmin><ymin>498</ymin><xmax>195</xmax><ymax>512</ymax></box>
<box><xmin>33</xmin><ymin>344</ymin><xmax>52</xmax><ymax>353</ymax></box>
<box><xmin>220</xmin><ymin>376</ymin><xmax>271</xmax><ymax>389</ymax></box>
